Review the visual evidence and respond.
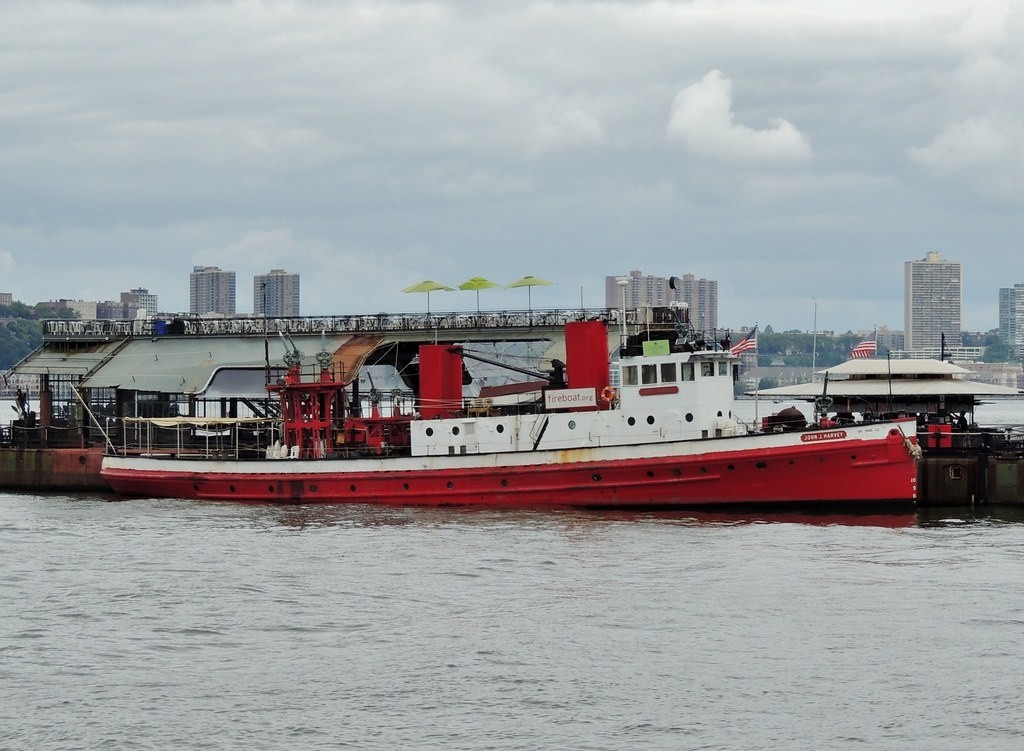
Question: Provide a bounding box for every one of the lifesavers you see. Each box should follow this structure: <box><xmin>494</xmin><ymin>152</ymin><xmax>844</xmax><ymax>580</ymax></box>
<box><xmin>601</xmin><ymin>386</ymin><xmax>615</xmax><ymax>401</ymax></box>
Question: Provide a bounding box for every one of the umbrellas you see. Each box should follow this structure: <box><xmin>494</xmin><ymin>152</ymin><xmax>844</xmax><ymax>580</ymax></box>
<box><xmin>400</xmin><ymin>279</ymin><xmax>456</xmax><ymax>312</ymax></box>
<box><xmin>504</xmin><ymin>275</ymin><xmax>555</xmax><ymax>309</ymax></box>
<box><xmin>457</xmin><ymin>277</ymin><xmax>499</xmax><ymax>311</ymax></box>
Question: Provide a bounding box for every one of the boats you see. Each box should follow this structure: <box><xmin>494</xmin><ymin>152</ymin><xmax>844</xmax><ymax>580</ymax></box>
<box><xmin>97</xmin><ymin>284</ymin><xmax>928</xmax><ymax>513</ymax></box>
<box><xmin>0</xmin><ymin>371</ymin><xmax>268</xmax><ymax>494</ymax></box>
<box><xmin>733</xmin><ymin>357</ymin><xmax>1024</xmax><ymax>506</ymax></box>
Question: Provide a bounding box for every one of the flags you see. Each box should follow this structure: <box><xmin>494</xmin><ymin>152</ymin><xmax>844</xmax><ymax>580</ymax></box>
<box><xmin>730</xmin><ymin>325</ymin><xmax>757</xmax><ymax>357</ymax></box>
<box><xmin>850</xmin><ymin>330</ymin><xmax>877</xmax><ymax>359</ymax></box>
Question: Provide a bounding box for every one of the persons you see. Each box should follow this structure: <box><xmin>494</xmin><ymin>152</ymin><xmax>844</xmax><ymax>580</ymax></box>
<box><xmin>955</xmin><ymin>411</ymin><xmax>972</xmax><ymax>448</ymax></box>
